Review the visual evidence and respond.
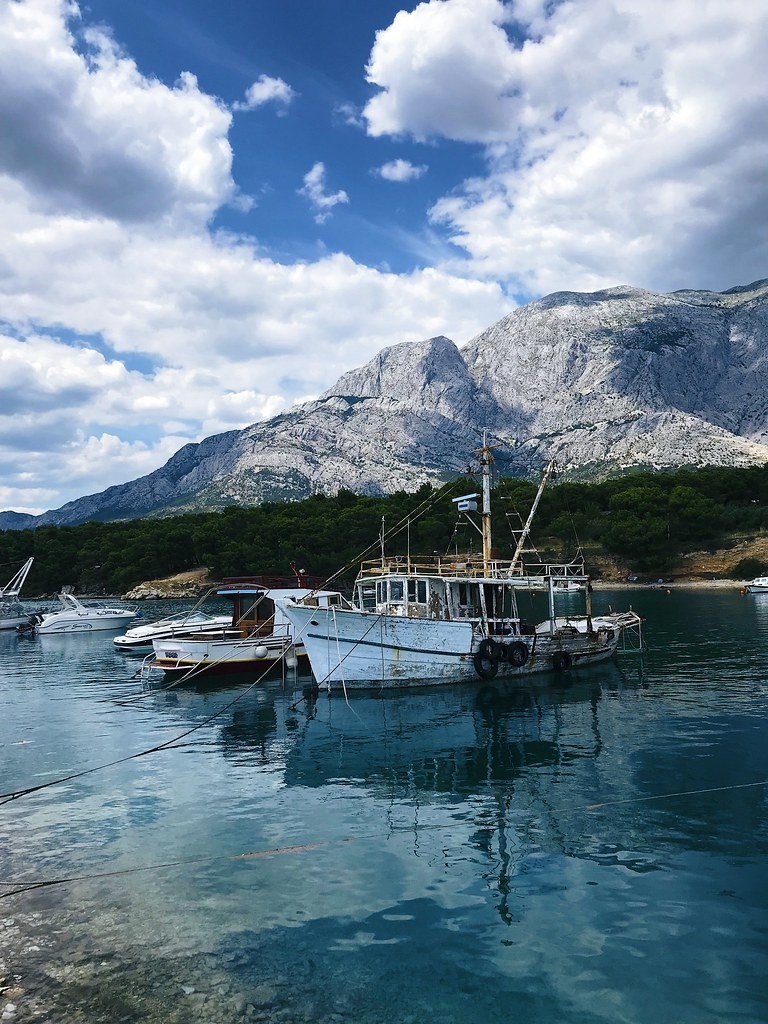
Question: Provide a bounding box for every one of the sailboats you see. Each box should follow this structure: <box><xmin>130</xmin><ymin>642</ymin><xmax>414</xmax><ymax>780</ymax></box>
<box><xmin>273</xmin><ymin>428</ymin><xmax>647</xmax><ymax>689</ymax></box>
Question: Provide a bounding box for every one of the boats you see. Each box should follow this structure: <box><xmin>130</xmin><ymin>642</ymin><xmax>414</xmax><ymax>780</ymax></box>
<box><xmin>112</xmin><ymin>610</ymin><xmax>234</xmax><ymax>657</ymax></box>
<box><xmin>133</xmin><ymin>582</ymin><xmax>353</xmax><ymax>677</ymax></box>
<box><xmin>744</xmin><ymin>577</ymin><xmax>768</xmax><ymax>593</ymax></box>
<box><xmin>0</xmin><ymin>555</ymin><xmax>140</xmax><ymax>634</ymax></box>
